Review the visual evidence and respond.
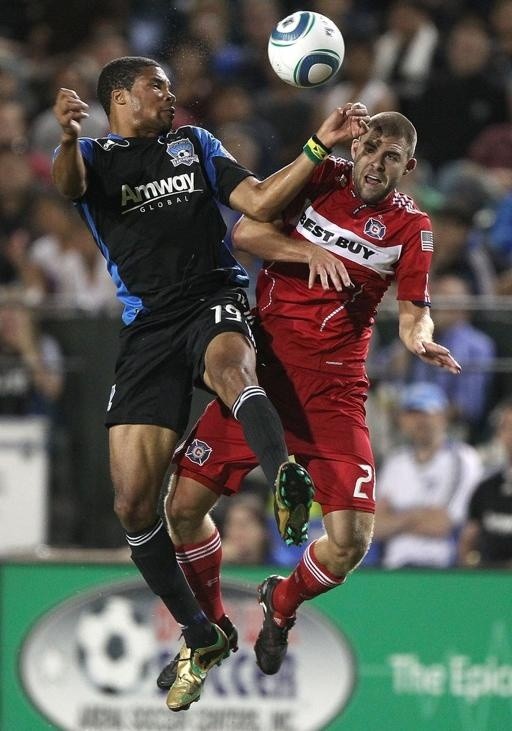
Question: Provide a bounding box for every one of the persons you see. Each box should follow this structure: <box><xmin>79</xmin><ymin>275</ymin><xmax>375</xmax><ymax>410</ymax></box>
<box><xmin>155</xmin><ymin>111</ymin><xmax>464</xmax><ymax>691</ymax></box>
<box><xmin>50</xmin><ymin>58</ymin><xmax>371</xmax><ymax>710</ymax></box>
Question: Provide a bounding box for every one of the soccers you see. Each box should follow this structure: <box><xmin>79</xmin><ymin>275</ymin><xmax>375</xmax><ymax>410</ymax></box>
<box><xmin>267</xmin><ymin>11</ymin><xmax>346</xmax><ymax>88</ymax></box>
<box><xmin>78</xmin><ymin>597</ymin><xmax>157</xmax><ymax>690</ymax></box>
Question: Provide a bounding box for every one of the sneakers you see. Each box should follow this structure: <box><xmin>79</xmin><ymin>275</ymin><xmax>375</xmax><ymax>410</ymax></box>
<box><xmin>273</xmin><ymin>461</ymin><xmax>315</xmax><ymax>548</ymax></box>
<box><xmin>254</xmin><ymin>571</ymin><xmax>298</xmax><ymax>676</ymax></box>
<box><xmin>157</xmin><ymin>615</ymin><xmax>238</xmax><ymax>712</ymax></box>
